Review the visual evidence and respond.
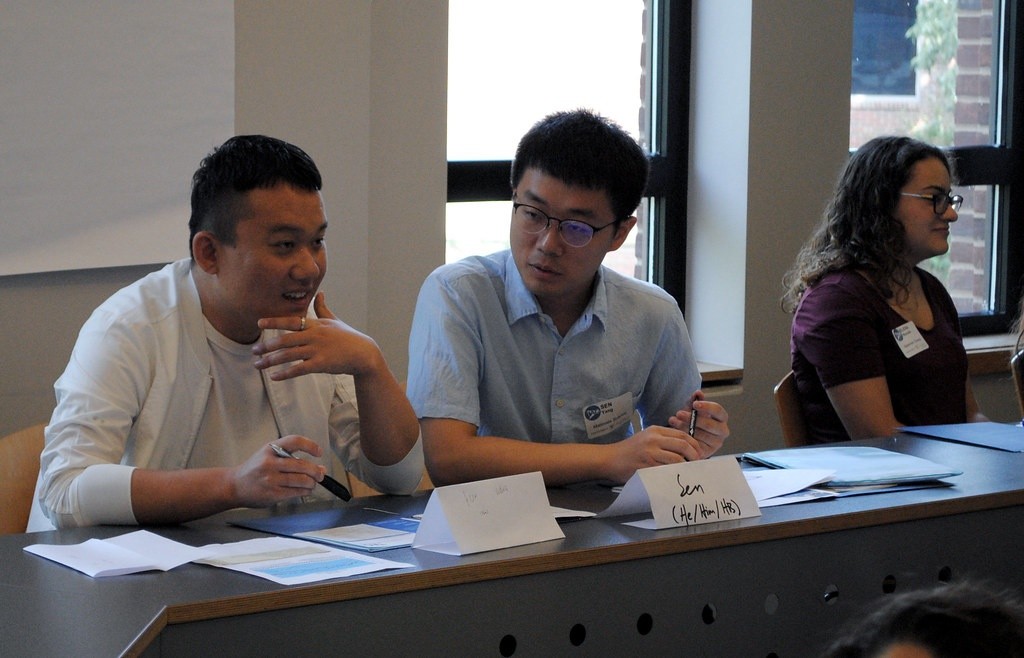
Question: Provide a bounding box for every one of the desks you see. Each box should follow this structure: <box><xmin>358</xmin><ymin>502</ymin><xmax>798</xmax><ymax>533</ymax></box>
<box><xmin>1</xmin><ymin>420</ymin><xmax>1023</xmax><ymax>658</ymax></box>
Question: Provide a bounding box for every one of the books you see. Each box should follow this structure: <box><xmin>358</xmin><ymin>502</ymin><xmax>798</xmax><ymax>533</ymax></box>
<box><xmin>226</xmin><ymin>505</ymin><xmax>421</xmax><ymax>552</ymax></box>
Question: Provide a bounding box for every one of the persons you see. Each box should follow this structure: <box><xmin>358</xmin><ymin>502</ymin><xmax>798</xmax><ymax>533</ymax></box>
<box><xmin>820</xmin><ymin>580</ymin><xmax>1024</xmax><ymax>658</ymax></box>
<box><xmin>406</xmin><ymin>113</ymin><xmax>729</xmax><ymax>488</ymax></box>
<box><xmin>780</xmin><ymin>137</ymin><xmax>989</xmax><ymax>445</ymax></box>
<box><xmin>38</xmin><ymin>134</ymin><xmax>425</xmax><ymax>529</ymax></box>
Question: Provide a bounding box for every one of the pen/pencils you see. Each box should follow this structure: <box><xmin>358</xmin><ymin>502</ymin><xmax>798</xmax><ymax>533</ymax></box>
<box><xmin>683</xmin><ymin>395</ymin><xmax>699</xmax><ymax>462</ymax></box>
<box><xmin>268</xmin><ymin>442</ymin><xmax>352</xmax><ymax>503</ymax></box>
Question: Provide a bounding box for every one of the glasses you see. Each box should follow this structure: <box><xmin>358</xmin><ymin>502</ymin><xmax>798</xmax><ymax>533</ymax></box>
<box><xmin>512</xmin><ymin>191</ymin><xmax>630</xmax><ymax>247</ymax></box>
<box><xmin>899</xmin><ymin>190</ymin><xmax>964</xmax><ymax>215</ymax></box>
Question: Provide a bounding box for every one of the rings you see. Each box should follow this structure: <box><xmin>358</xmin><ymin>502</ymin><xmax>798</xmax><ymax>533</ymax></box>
<box><xmin>300</xmin><ymin>316</ymin><xmax>305</xmax><ymax>331</ymax></box>
<box><xmin>284</xmin><ymin>472</ymin><xmax>289</xmax><ymax>487</ymax></box>
